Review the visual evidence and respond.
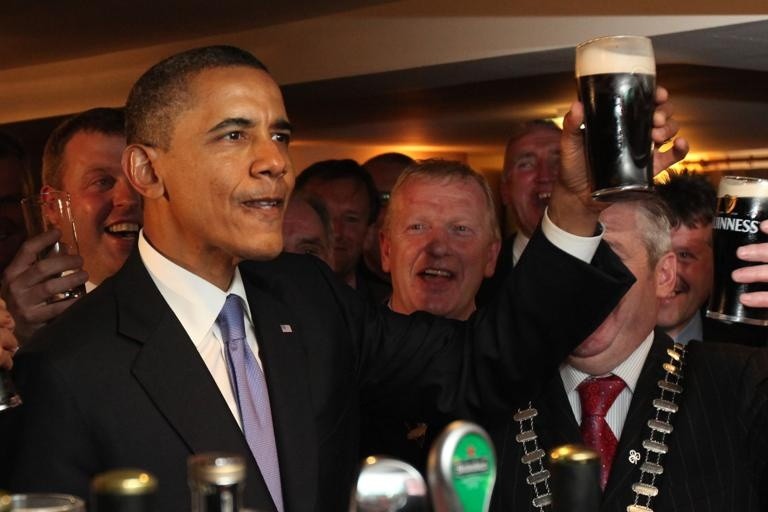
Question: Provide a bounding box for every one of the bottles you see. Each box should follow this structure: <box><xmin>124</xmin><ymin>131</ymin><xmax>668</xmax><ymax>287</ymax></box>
<box><xmin>190</xmin><ymin>452</ymin><xmax>248</xmax><ymax>512</ymax></box>
<box><xmin>554</xmin><ymin>442</ymin><xmax>599</xmax><ymax>511</ymax></box>
<box><xmin>90</xmin><ymin>467</ymin><xmax>157</xmax><ymax>510</ymax></box>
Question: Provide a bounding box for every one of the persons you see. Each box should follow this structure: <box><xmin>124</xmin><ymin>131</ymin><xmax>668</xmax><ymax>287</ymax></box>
<box><xmin>0</xmin><ymin>107</ymin><xmax>145</xmax><ymax>350</ymax></box>
<box><xmin>0</xmin><ymin>45</ymin><xmax>689</xmax><ymax>512</ymax></box>
<box><xmin>276</xmin><ymin>120</ymin><xmax>768</xmax><ymax>512</ymax></box>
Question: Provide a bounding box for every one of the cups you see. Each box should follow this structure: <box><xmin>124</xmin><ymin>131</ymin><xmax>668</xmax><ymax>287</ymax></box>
<box><xmin>22</xmin><ymin>190</ymin><xmax>86</xmax><ymax>303</ymax></box>
<box><xmin>577</xmin><ymin>36</ymin><xmax>656</xmax><ymax>201</ymax></box>
<box><xmin>0</xmin><ymin>494</ymin><xmax>83</xmax><ymax>512</ymax></box>
<box><xmin>706</xmin><ymin>175</ymin><xmax>768</xmax><ymax>327</ymax></box>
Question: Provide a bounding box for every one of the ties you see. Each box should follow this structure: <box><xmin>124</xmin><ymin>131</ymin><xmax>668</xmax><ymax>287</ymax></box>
<box><xmin>216</xmin><ymin>295</ymin><xmax>286</xmax><ymax>512</ymax></box>
<box><xmin>575</xmin><ymin>374</ymin><xmax>627</xmax><ymax>490</ymax></box>
